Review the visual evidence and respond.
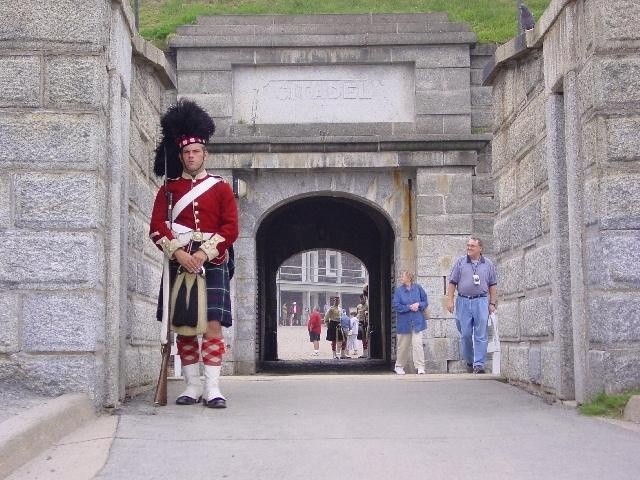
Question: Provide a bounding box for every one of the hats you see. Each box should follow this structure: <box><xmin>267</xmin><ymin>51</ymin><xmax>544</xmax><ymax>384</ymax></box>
<box><xmin>152</xmin><ymin>100</ymin><xmax>215</xmax><ymax>178</ymax></box>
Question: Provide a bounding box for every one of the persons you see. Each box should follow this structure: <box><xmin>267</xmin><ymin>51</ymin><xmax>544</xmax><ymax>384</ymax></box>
<box><xmin>446</xmin><ymin>236</ymin><xmax>498</xmax><ymax>376</ymax></box>
<box><xmin>280</xmin><ymin>283</ymin><xmax>371</xmax><ymax>360</ymax></box>
<box><xmin>146</xmin><ymin>137</ymin><xmax>241</xmax><ymax>410</ymax></box>
<box><xmin>388</xmin><ymin>267</ymin><xmax>429</xmax><ymax>376</ymax></box>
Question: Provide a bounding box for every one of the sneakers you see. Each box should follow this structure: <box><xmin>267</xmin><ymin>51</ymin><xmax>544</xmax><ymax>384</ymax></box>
<box><xmin>417</xmin><ymin>368</ymin><xmax>426</xmax><ymax>374</ymax></box>
<box><xmin>472</xmin><ymin>365</ymin><xmax>485</xmax><ymax>374</ymax></box>
<box><xmin>333</xmin><ymin>355</ymin><xmax>339</xmax><ymax>359</ymax></box>
<box><xmin>466</xmin><ymin>363</ymin><xmax>473</xmax><ymax>372</ymax></box>
<box><xmin>340</xmin><ymin>355</ymin><xmax>351</xmax><ymax>359</ymax></box>
<box><xmin>394</xmin><ymin>365</ymin><xmax>405</xmax><ymax>374</ymax></box>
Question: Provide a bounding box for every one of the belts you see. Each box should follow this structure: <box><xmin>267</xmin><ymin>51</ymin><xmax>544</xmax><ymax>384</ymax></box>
<box><xmin>191</xmin><ymin>232</ymin><xmax>213</xmax><ymax>241</ymax></box>
<box><xmin>458</xmin><ymin>293</ymin><xmax>486</xmax><ymax>299</ymax></box>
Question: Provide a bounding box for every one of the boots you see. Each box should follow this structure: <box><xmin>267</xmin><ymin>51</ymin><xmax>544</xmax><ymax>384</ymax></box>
<box><xmin>176</xmin><ymin>360</ymin><xmax>203</xmax><ymax>404</ymax></box>
<box><xmin>201</xmin><ymin>364</ymin><xmax>227</xmax><ymax>408</ymax></box>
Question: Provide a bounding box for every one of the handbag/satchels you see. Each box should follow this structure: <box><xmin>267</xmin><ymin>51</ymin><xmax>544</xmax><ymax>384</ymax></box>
<box><xmin>423</xmin><ymin>309</ymin><xmax>431</xmax><ymax>321</ymax></box>
<box><xmin>168</xmin><ymin>265</ymin><xmax>207</xmax><ymax>337</ymax></box>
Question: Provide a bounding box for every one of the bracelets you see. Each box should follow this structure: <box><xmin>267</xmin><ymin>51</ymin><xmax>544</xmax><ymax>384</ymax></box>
<box><xmin>490</xmin><ymin>302</ymin><xmax>496</xmax><ymax>308</ymax></box>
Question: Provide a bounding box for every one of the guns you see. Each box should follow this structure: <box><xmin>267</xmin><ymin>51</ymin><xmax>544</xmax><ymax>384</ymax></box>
<box><xmin>155</xmin><ymin>148</ymin><xmax>174</xmax><ymax>407</ymax></box>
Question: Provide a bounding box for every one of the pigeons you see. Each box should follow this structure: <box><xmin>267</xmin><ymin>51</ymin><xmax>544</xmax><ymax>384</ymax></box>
<box><xmin>518</xmin><ymin>4</ymin><xmax>535</xmax><ymax>31</ymax></box>
